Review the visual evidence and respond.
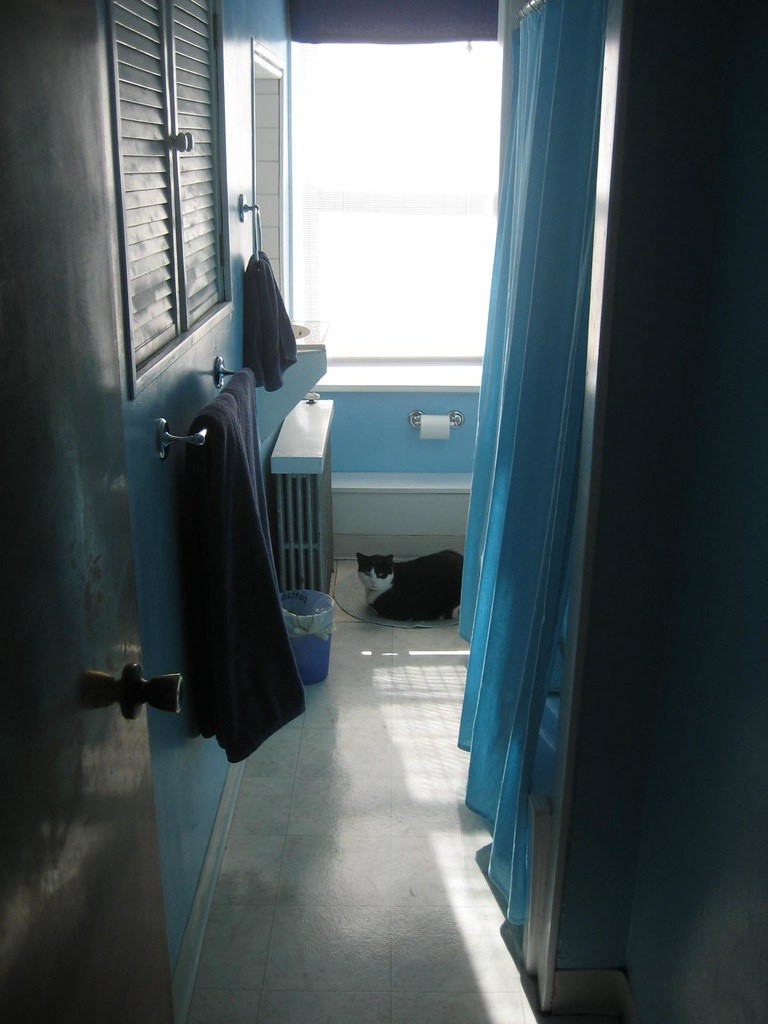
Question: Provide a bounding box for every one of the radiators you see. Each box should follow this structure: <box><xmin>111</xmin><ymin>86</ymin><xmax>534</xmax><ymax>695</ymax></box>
<box><xmin>269</xmin><ymin>427</ymin><xmax>334</xmax><ymax>594</ymax></box>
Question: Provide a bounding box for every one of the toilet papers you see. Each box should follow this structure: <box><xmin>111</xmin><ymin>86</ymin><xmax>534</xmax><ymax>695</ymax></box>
<box><xmin>419</xmin><ymin>414</ymin><xmax>451</xmax><ymax>440</ymax></box>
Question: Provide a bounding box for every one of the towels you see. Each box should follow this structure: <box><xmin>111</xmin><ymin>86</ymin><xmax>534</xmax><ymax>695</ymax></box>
<box><xmin>243</xmin><ymin>251</ymin><xmax>298</xmax><ymax>393</ymax></box>
<box><xmin>175</xmin><ymin>367</ymin><xmax>307</xmax><ymax>764</ymax></box>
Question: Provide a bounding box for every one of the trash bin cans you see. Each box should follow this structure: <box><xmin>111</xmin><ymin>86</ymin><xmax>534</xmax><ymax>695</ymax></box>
<box><xmin>278</xmin><ymin>589</ymin><xmax>334</xmax><ymax>687</ymax></box>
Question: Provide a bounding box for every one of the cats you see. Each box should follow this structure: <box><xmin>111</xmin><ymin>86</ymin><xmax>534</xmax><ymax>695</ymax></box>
<box><xmin>354</xmin><ymin>550</ymin><xmax>464</xmax><ymax>622</ymax></box>
